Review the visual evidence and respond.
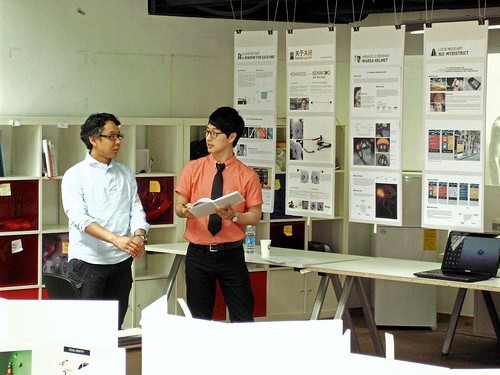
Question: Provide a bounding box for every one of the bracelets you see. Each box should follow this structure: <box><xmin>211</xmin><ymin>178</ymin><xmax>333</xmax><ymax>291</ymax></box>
<box><xmin>135</xmin><ymin>234</ymin><xmax>144</xmax><ymax>240</ymax></box>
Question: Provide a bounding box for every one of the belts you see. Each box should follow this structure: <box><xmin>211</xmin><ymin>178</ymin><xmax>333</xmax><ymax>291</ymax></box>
<box><xmin>190</xmin><ymin>240</ymin><xmax>242</xmax><ymax>252</ymax></box>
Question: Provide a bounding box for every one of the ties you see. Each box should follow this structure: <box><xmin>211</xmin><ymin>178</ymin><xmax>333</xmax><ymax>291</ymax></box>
<box><xmin>208</xmin><ymin>163</ymin><xmax>225</xmax><ymax>236</ymax></box>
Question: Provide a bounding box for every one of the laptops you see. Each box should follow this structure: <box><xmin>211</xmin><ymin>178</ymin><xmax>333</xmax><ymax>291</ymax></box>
<box><xmin>413</xmin><ymin>230</ymin><xmax>500</xmax><ymax>282</ymax></box>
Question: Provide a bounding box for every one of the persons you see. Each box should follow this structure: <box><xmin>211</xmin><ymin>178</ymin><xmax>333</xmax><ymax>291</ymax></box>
<box><xmin>357</xmin><ymin>56</ymin><xmax>361</xmax><ymax>63</ymax></box>
<box><xmin>354</xmin><ymin>87</ymin><xmax>361</xmax><ymax>107</ymax></box>
<box><xmin>175</xmin><ymin>107</ymin><xmax>263</xmax><ymax>323</ymax></box>
<box><xmin>301</xmin><ymin>98</ymin><xmax>307</xmax><ymax>110</ymax></box>
<box><xmin>433</xmin><ymin>103</ymin><xmax>442</xmax><ymax>112</ymax></box>
<box><xmin>61</xmin><ymin>113</ymin><xmax>150</xmax><ymax>330</ymax></box>
<box><xmin>489</xmin><ymin>116</ymin><xmax>500</xmax><ymax>185</ymax></box>
<box><xmin>237</xmin><ymin>144</ymin><xmax>248</xmax><ymax>156</ymax></box>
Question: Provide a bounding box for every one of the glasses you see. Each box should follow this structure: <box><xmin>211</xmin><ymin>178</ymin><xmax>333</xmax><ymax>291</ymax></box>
<box><xmin>204</xmin><ymin>129</ymin><xmax>224</xmax><ymax>138</ymax></box>
<box><xmin>99</xmin><ymin>134</ymin><xmax>124</xmax><ymax>140</ymax></box>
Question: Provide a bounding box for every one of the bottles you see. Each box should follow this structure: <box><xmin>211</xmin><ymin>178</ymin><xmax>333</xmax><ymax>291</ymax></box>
<box><xmin>245</xmin><ymin>225</ymin><xmax>255</xmax><ymax>255</ymax></box>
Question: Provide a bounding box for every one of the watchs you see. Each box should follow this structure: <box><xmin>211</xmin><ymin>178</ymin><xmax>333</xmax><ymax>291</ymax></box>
<box><xmin>231</xmin><ymin>212</ymin><xmax>238</xmax><ymax>222</ymax></box>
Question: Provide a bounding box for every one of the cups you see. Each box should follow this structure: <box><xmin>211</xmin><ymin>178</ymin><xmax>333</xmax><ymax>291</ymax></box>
<box><xmin>260</xmin><ymin>239</ymin><xmax>272</xmax><ymax>257</ymax></box>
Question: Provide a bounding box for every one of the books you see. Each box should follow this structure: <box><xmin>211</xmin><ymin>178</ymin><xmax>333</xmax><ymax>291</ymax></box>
<box><xmin>42</xmin><ymin>139</ymin><xmax>57</xmax><ymax>178</ymax></box>
<box><xmin>187</xmin><ymin>191</ymin><xmax>245</xmax><ymax>219</ymax></box>
<box><xmin>0</xmin><ymin>137</ymin><xmax>6</xmax><ymax>177</ymax></box>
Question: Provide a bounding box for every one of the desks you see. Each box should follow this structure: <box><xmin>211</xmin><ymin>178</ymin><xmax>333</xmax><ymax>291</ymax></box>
<box><xmin>307</xmin><ymin>257</ymin><xmax>500</xmax><ymax>359</ymax></box>
<box><xmin>142</xmin><ymin>242</ymin><xmax>361</xmax><ymax>353</ymax></box>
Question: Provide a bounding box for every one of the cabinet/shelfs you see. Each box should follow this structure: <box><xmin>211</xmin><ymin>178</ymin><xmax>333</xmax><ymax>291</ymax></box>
<box><xmin>184</xmin><ymin>118</ymin><xmax>348</xmax><ymax>321</ymax></box>
<box><xmin>0</xmin><ymin>117</ymin><xmax>184</xmax><ymax>327</ymax></box>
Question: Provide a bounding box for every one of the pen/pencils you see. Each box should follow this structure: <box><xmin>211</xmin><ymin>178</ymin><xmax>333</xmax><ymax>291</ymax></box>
<box><xmin>179</xmin><ymin>201</ymin><xmax>187</xmax><ymax>208</ymax></box>
